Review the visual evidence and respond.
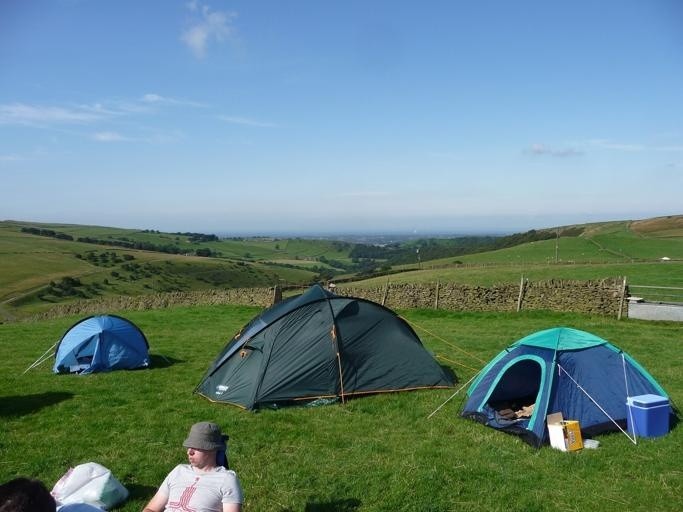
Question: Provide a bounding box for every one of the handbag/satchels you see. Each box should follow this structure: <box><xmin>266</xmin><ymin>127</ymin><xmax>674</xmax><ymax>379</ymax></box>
<box><xmin>499</xmin><ymin>409</ymin><xmax>515</xmax><ymax>418</ymax></box>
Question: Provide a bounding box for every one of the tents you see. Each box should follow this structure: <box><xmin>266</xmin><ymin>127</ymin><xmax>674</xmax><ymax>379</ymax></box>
<box><xmin>51</xmin><ymin>313</ymin><xmax>152</xmax><ymax>373</ymax></box>
<box><xmin>193</xmin><ymin>283</ymin><xmax>453</xmax><ymax>410</ymax></box>
<box><xmin>459</xmin><ymin>325</ymin><xmax>676</xmax><ymax>449</ymax></box>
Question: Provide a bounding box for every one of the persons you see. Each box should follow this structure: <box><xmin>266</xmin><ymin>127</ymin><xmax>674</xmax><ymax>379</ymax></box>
<box><xmin>140</xmin><ymin>423</ymin><xmax>243</xmax><ymax>511</ymax></box>
<box><xmin>1</xmin><ymin>477</ymin><xmax>105</xmax><ymax>512</ymax></box>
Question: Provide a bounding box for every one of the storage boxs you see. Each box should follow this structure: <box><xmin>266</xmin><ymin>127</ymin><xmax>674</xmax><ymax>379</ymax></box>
<box><xmin>626</xmin><ymin>394</ymin><xmax>669</xmax><ymax>438</ymax></box>
<box><xmin>547</xmin><ymin>411</ymin><xmax>583</xmax><ymax>452</ymax></box>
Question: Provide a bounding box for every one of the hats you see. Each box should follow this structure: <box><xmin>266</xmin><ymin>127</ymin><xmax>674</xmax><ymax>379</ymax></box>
<box><xmin>182</xmin><ymin>422</ymin><xmax>227</xmax><ymax>450</ymax></box>
